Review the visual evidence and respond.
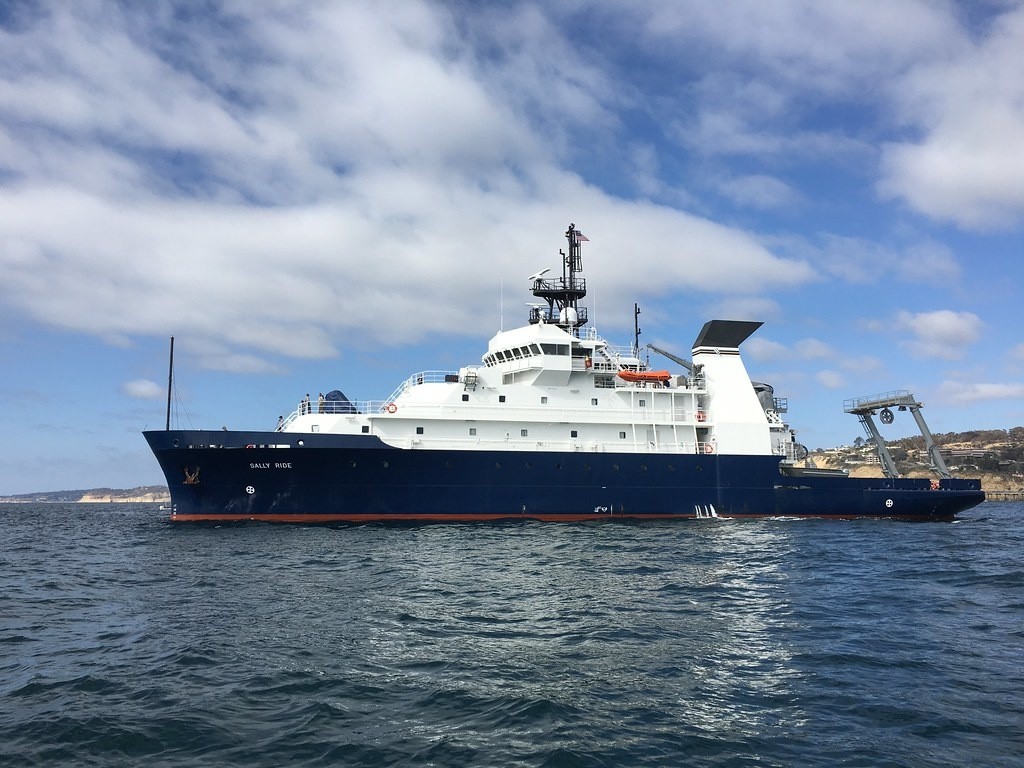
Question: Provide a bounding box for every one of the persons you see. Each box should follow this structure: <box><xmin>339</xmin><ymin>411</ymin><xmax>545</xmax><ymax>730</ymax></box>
<box><xmin>305</xmin><ymin>394</ymin><xmax>311</xmax><ymax>414</ymax></box>
<box><xmin>300</xmin><ymin>400</ymin><xmax>305</xmax><ymax>415</ymax></box>
<box><xmin>318</xmin><ymin>393</ymin><xmax>324</xmax><ymax>413</ymax></box>
<box><xmin>278</xmin><ymin>415</ymin><xmax>283</xmax><ymax>430</ymax></box>
<box><xmin>417</xmin><ymin>374</ymin><xmax>422</xmax><ymax>384</ymax></box>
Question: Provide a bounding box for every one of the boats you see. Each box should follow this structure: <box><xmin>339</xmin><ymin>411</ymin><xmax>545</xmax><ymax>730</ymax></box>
<box><xmin>141</xmin><ymin>220</ymin><xmax>985</xmax><ymax>522</ymax></box>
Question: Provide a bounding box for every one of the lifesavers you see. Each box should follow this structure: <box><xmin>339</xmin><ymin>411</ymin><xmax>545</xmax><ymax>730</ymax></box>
<box><xmin>388</xmin><ymin>403</ymin><xmax>397</xmax><ymax>413</ymax></box>
<box><xmin>705</xmin><ymin>444</ymin><xmax>713</xmax><ymax>454</ymax></box>
<box><xmin>697</xmin><ymin>412</ymin><xmax>706</xmax><ymax>421</ymax></box>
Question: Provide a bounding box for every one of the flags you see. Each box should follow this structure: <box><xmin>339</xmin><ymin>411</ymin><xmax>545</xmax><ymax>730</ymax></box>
<box><xmin>577</xmin><ymin>233</ymin><xmax>589</xmax><ymax>241</ymax></box>
